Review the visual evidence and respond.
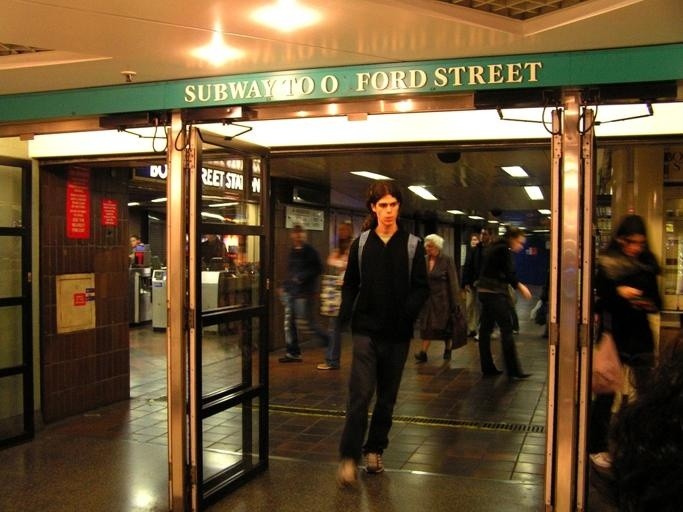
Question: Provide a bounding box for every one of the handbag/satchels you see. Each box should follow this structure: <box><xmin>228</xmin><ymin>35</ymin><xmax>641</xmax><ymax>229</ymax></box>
<box><xmin>449</xmin><ymin>310</ymin><xmax>468</xmax><ymax>351</ymax></box>
<box><xmin>319</xmin><ymin>272</ymin><xmax>344</xmax><ymax>318</ymax></box>
<box><xmin>591</xmin><ymin>330</ymin><xmax>623</xmax><ymax>395</ymax></box>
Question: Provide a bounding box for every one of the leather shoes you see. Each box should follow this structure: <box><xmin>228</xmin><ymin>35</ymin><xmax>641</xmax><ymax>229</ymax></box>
<box><xmin>516</xmin><ymin>372</ymin><xmax>531</xmax><ymax>378</ymax></box>
<box><xmin>482</xmin><ymin>368</ymin><xmax>503</xmax><ymax>377</ymax></box>
<box><xmin>443</xmin><ymin>348</ymin><xmax>451</xmax><ymax>360</ymax></box>
<box><xmin>413</xmin><ymin>351</ymin><xmax>428</xmax><ymax>361</ymax></box>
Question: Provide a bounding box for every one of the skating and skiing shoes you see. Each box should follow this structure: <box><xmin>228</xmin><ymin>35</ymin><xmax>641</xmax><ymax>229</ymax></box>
<box><xmin>589</xmin><ymin>451</ymin><xmax>612</xmax><ymax>469</ymax></box>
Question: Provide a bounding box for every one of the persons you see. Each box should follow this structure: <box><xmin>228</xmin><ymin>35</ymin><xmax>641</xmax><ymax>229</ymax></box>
<box><xmin>414</xmin><ymin>223</ymin><xmax>551</xmax><ymax>383</ymax></box>
<box><xmin>275</xmin><ymin>221</ymin><xmax>354</xmax><ymax>370</ymax></box>
<box><xmin>333</xmin><ymin>180</ymin><xmax>431</xmax><ymax>488</ymax></box>
<box><xmin>129</xmin><ymin>235</ymin><xmax>149</xmax><ymax>288</ymax></box>
<box><xmin>585</xmin><ymin>214</ymin><xmax>682</xmax><ymax>511</ymax></box>
<box><xmin>200</xmin><ymin>234</ymin><xmax>229</xmax><ymax>271</ymax></box>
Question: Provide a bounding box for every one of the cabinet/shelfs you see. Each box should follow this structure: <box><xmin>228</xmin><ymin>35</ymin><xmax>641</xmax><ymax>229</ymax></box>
<box><xmin>632</xmin><ymin>143</ymin><xmax>683</xmax><ymax>329</ymax></box>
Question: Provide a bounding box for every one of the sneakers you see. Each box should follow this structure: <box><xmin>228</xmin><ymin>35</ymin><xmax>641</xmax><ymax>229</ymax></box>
<box><xmin>277</xmin><ymin>356</ymin><xmax>303</xmax><ymax>363</ymax></box>
<box><xmin>363</xmin><ymin>451</ymin><xmax>384</xmax><ymax>473</ymax></box>
<box><xmin>334</xmin><ymin>459</ymin><xmax>359</xmax><ymax>491</ymax></box>
<box><xmin>317</xmin><ymin>364</ymin><xmax>330</xmax><ymax>370</ymax></box>
